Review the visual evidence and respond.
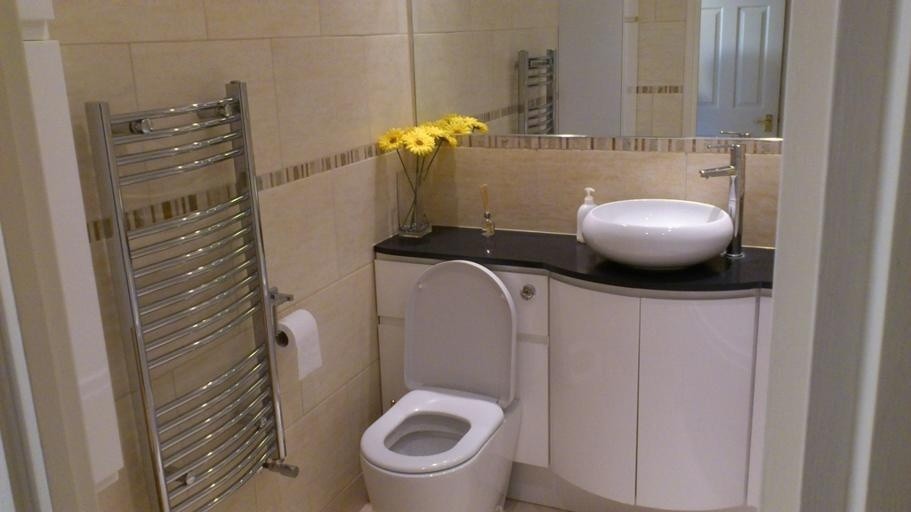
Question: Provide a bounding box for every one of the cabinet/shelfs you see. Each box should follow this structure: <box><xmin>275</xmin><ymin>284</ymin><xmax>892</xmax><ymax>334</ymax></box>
<box><xmin>481</xmin><ymin>213</ymin><xmax>496</xmax><ymax>237</ymax></box>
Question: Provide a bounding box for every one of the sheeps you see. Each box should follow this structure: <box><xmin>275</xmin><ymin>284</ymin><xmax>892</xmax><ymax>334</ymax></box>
<box><xmin>697</xmin><ymin>144</ymin><xmax>748</xmax><ymax>261</ymax></box>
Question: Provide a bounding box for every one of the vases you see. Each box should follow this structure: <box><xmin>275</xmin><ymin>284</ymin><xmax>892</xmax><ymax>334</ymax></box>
<box><xmin>378</xmin><ymin>124</ymin><xmax>461</xmax><ymax>230</ymax></box>
<box><xmin>439</xmin><ymin>113</ymin><xmax>493</xmax><ymax>136</ymax></box>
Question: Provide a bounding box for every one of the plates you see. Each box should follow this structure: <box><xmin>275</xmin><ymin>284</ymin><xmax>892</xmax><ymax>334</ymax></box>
<box><xmin>549</xmin><ymin>273</ymin><xmax>772</xmax><ymax>512</ymax></box>
<box><xmin>368</xmin><ymin>244</ymin><xmax>551</xmax><ymax>504</ymax></box>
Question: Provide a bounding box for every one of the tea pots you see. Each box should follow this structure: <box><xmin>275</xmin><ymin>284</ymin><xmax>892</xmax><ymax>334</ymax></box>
<box><xmin>409</xmin><ymin>2</ymin><xmax>787</xmax><ymax>155</ymax></box>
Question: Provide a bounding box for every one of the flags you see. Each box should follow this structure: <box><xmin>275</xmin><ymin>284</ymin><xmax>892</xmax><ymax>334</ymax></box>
<box><xmin>393</xmin><ymin>171</ymin><xmax>432</xmax><ymax>238</ymax></box>
